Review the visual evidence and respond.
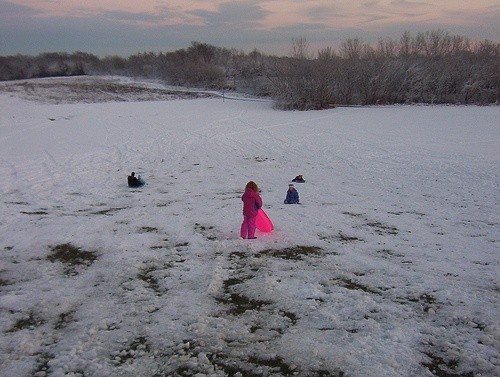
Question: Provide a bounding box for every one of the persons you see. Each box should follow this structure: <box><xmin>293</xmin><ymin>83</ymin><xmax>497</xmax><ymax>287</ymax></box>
<box><xmin>138</xmin><ymin>174</ymin><xmax>145</xmax><ymax>185</ymax></box>
<box><xmin>240</xmin><ymin>180</ymin><xmax>263</xmax><ymax>239</ymax></box>
<box><xmin>292</xmin><ymin>174</ymin><xmax>305</xmax><ymax>184</ymax></box>
<box><xmin>127</xmin><ymin>172</ymin><xmax>143</xmax><ymax>187</ymax></box>
<box><xmin>283</xmin><ymin>184</ymin><xmax>301</xmax><ymax>204</ymax></box>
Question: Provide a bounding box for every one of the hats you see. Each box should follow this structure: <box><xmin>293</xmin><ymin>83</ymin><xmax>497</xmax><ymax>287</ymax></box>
<box><xmin>289</xmin><ymin>184</ymin><xmax>294</xmax><ymax>187</ymax></box>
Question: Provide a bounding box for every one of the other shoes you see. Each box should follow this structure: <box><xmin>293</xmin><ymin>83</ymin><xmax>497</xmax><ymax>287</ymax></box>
<box><xmin>248</xmin><ymin>237</ymin><xmax>257</xmax><ymax>239</ymax></box>
